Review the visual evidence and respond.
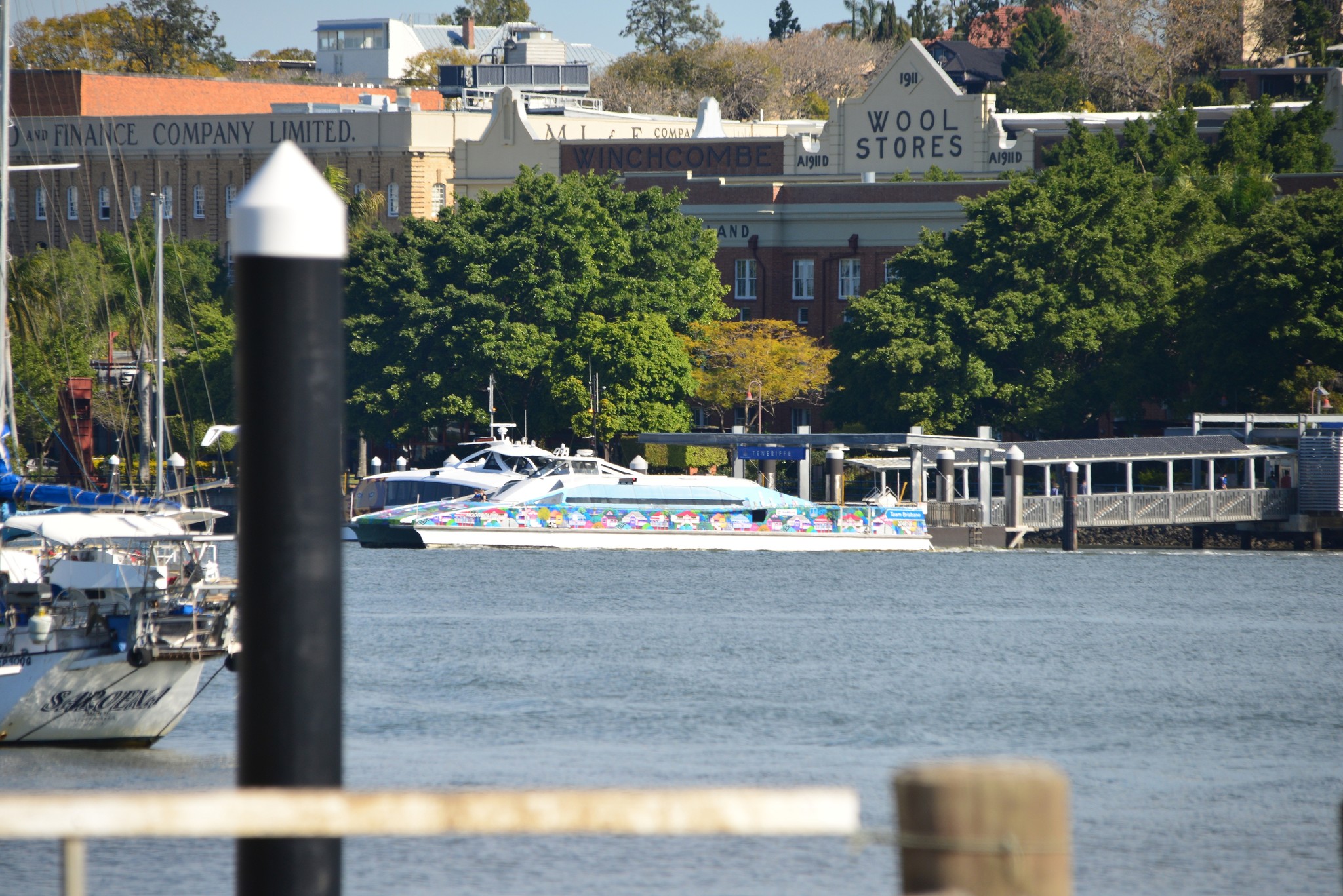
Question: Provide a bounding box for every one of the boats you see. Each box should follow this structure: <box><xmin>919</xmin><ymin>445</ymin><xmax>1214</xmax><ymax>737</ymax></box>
<box><xmin>345</xmin><ymin>443</ymin><xmax>935</xmax><ymax>554</ymax></box>
<box><xmin>349</xmin><ymin>372</ymin><xmax>569</xmax><ymax>516</ymax></box>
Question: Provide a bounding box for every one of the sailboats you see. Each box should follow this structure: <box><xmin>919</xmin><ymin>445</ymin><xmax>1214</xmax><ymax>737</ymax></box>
<box><xmin>1</xmin><ymin>162</ymin><xmax>247</xmax><ymax>748</ymax></box>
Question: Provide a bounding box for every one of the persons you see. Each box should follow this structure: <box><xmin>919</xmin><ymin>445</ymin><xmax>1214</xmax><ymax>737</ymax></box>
<box><xmin>1265</xmin><ymin>470</ymin><xmax>1278</xmax><ymax>489</ymax></box>
<box><xmin>1218</xmin><ymin>472</ymin><xmax>1228</xmax><ymax>490</ymax></box>
<box><xmin>1281</xmin><ymin>470</ymin><xmax>1291</xmax><ymax>489</ymax></box>
<box><xmin>1077</xmin><ymin>481</ymin><xmax>1087</xmax><ymax>495</ymax></box>
<box><xmin>1051</xmin><ymin>483</ymin><xmax>1059</xmax><ymax>496</ymax></box>
